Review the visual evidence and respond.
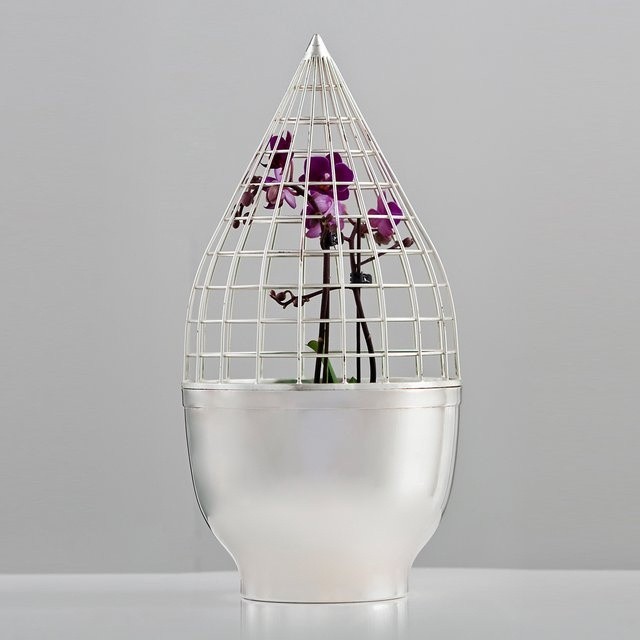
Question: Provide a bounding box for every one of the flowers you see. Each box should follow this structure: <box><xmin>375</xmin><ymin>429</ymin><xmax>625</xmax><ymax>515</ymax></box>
<box><xmin>230</xmin><ymin>131</ymin><xmax>414</xmax><ymax>384</ymax></box>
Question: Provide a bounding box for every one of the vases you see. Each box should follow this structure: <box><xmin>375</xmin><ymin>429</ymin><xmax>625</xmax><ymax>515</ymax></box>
<box><xmin>182</xmin><ymin>34</ymin><xmax>460</xmax><ymax>605</ymax></box>
